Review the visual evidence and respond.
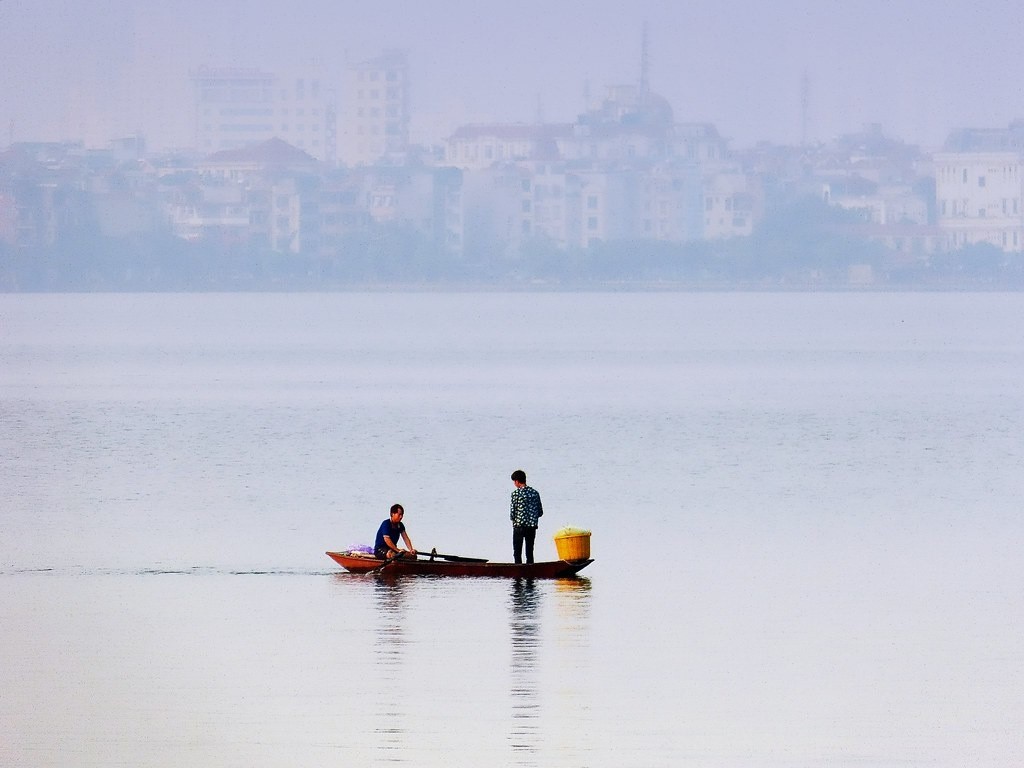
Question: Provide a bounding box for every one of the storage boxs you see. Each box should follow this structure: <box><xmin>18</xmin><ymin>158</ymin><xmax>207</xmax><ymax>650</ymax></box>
<box><xmin>554</xmin><ymin>532</ymin><xmax>591</xmax><ymax>561</ymax></box>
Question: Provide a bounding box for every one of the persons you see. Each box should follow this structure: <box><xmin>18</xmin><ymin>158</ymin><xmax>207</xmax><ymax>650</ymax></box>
<box><xmin>510</xmin><ymin>470</ymin><xmax>544</xmax><ymax>564</ymax></box>
<box><xmin>374</xmin><ymin>504</ymin><xmax>418</xmax><ymax>560</ymax></box>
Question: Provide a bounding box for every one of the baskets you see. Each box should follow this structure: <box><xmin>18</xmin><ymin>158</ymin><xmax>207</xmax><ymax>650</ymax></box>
<box><xmin>553</xmin><ymin>533</ymin><xmax>592</xmax><ymax>561</ymax></box>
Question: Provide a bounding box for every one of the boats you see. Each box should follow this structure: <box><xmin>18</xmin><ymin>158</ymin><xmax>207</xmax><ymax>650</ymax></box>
<box><xmin>326</xmin><ymin>551</ymin><xmax>597</xmax><ymax>577</ymax></box>
